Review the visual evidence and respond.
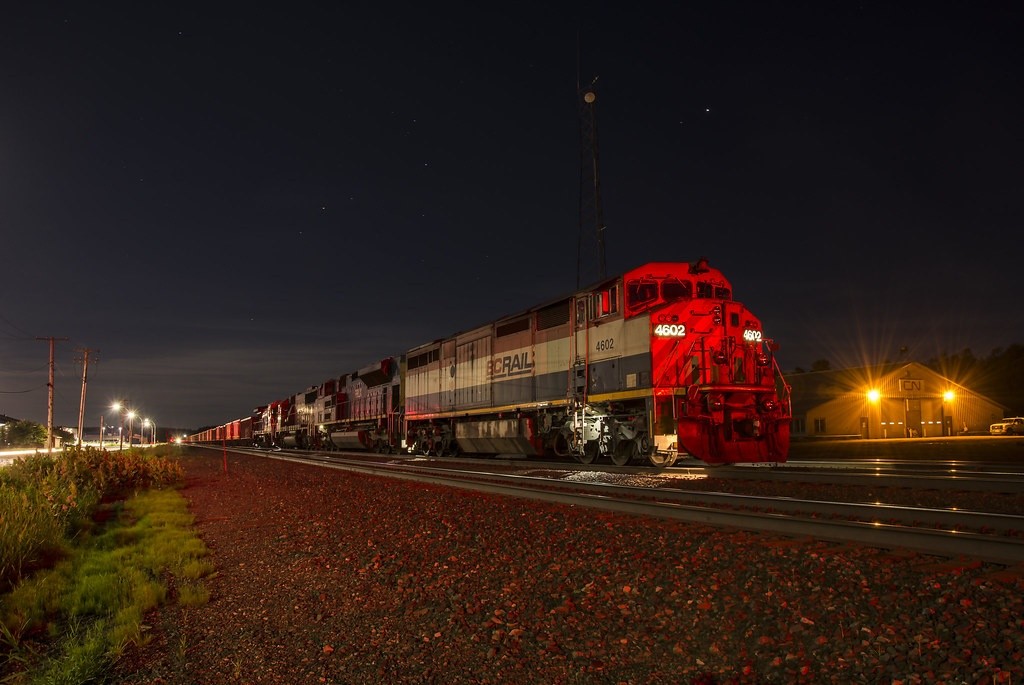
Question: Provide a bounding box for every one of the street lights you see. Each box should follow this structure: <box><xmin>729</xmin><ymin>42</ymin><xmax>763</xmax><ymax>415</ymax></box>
<box><xmin>142</xmin><ymin>418</ymin><xmax>156</xmax><ymax>444</ymax></box>
<box><xmin>111</xmin><ymin>401</ymin><xmax>124</xmax><ymax>450</ymax></box>
<box><xmin>143</xmin><ymin>423</ymin><xmax>153</xmax><ymax>442</ymax></box>
<box><xmin>130</xmin><ymin>410</ymin><xmax>143</xmax><ymax>446</ymax></box>
<box><xmin>126</xmin><ymin>408</ymin><xmax>136</xmax><ymax>447</ymax></box>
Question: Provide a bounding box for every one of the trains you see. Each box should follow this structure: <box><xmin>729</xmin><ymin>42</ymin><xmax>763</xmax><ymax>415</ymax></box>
<box><xmin>169</xmin><ymin>436</ymin><xmax>188</xmax><ymax>444</ymax></box>
<box><xmin>189</xmin><ymin>257</ymin><xmax>792</xmax><ymax>468</ymax></box>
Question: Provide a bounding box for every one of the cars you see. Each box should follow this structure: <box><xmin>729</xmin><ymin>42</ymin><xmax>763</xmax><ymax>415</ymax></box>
<box><xmin>990</xmin><ymin>416</ymin><xmax>1024</xmax><ymax>436</ymax></box>
